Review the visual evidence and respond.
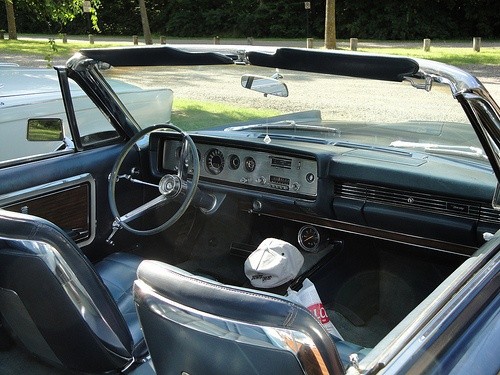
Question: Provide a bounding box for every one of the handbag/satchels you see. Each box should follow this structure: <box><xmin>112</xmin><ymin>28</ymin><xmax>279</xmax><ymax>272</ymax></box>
<box><xmin>286</xmin><ymin>279</ymin><xmax>344</xmax><ymax>342</ymax></box>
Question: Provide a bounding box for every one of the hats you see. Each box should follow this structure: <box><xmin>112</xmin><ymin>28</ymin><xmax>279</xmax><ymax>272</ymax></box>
<box><xmin>243</xmin><ymin>237</ymin><xmax>304</xmax><ymax>288</ymax></box>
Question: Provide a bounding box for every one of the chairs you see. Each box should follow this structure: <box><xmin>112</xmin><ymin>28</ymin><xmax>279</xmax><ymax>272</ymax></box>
<box><xmin>133</xmin><ymin>259</ymin><xmax>373</xmax><ymax>375</ymax></box>
<box><xmin>0</xmin><ymin>207</ymin><xmax>149</xmax><ymax>375</ymax></box>
